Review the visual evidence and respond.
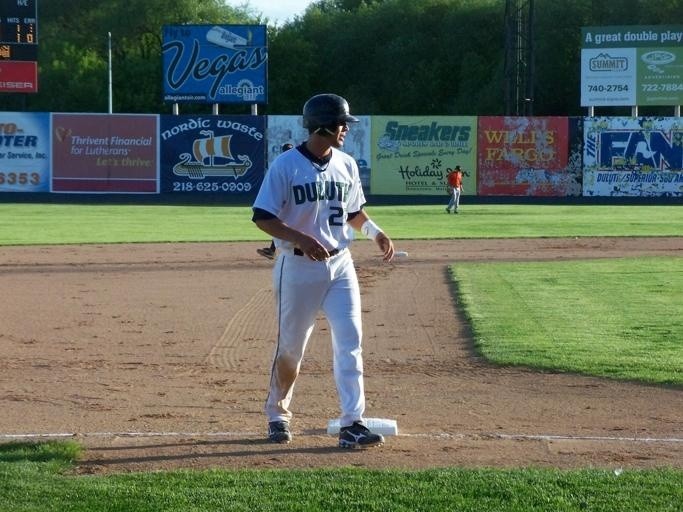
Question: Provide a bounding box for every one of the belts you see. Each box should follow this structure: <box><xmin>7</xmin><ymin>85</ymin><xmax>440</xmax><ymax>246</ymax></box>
<box><xmin>293</xmin><ymin>246</ymin><xmax>341</xmax><ymax>259</ymax></box>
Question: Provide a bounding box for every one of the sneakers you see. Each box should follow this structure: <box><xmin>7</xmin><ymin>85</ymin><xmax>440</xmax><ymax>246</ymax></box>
<box><xmin>337</xmin><ymin>420</ymin><xmax>385</xmax><ymax>449</ymax></box>
<box><xmin>266</xmin><ymin>419</ymin><xmax>292</xmax><ymax>445</ymax></box>
<box><xmin>445</xmin><ymin>208</ymin><xmax>458</xmax><ymax>214</ymax></box>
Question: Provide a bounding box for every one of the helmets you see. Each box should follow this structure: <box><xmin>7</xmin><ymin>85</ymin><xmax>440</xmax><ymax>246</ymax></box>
<box><xmin>300</xmin><ymin>93</ymin><xmax>360</xmax><ymax>127</ymax></box>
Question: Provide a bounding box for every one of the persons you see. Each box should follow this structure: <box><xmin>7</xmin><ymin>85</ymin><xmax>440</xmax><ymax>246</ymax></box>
<box><xmin>444</xmin><ymin>165</ymin><xmax>465</xmax><ymax>214</ymax></box>
<box><xmin>250</xmin><ymin>93</ymin><xmax>396</xmax><ymax>450</ymax></box>
<box><xmin>256</xmin><ymin>143</ymin><xmax>293</xmax><ymax>261</ymax></box>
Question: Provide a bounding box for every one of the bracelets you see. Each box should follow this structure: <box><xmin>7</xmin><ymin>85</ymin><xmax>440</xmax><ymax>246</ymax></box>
<box><xmin>360</xmin><ymin>220</ymin><xmax>384</xmax><ymax>241</ymax></box>
